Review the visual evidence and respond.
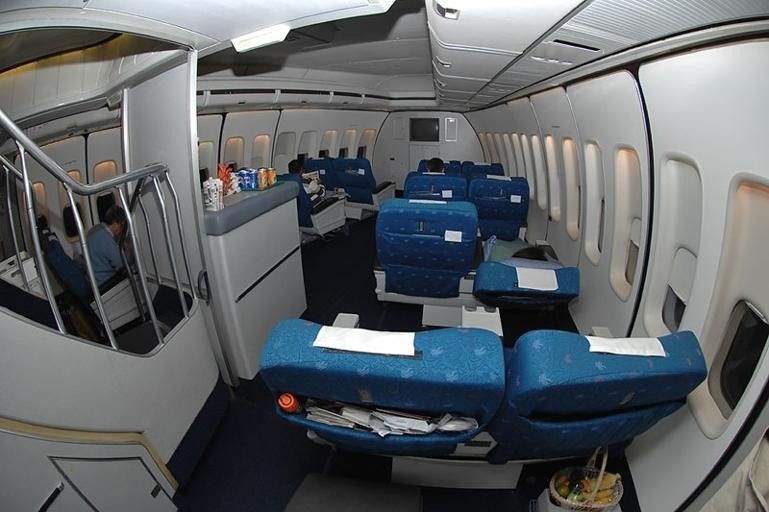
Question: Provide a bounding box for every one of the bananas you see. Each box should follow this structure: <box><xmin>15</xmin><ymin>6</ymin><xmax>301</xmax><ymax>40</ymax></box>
<box><xmin>568</xmin><ymin>488</ymin><xmax>614</xmax><ymax>503</ymax></box>
<box><xmin>579</xmin><ymin>473</ymin><xmax>622</xmax><ymax>492</ymax></box>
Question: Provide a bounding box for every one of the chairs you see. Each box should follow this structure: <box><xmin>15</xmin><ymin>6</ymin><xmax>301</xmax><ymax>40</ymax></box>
<box><xmin>257</xmin><ymin>157</ymin><xmax>707</xmax><ymax>472</ymax></box>
<box><xmin>36</xmin><ymin>223</ymin><xmax>159</xmax><ymax>332</ymax></box>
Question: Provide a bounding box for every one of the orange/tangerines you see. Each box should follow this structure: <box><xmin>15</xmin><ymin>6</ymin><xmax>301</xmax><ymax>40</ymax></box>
<box><xmin>557</xmin><ymin>485</ymin><xmax>569</xmax><ymax>495</ymax></box>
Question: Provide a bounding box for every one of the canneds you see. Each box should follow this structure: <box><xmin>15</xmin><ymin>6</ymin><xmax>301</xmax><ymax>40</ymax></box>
<box><xmin>235</xmin><ymin>166</ymin><xmax>278</xmax><ymax>190</ymax></box>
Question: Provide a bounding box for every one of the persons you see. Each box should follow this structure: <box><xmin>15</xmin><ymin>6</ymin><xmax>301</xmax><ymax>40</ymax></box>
<box><xmin>288</xmin><ymin>159</ymin><xmax>343</xmax><ymax>234</ymax></box>
<box><xmin>84</xmin><ymin>206</ymin><xmax>127</xmax><ymax>287</ymax></box>
<box><xmin>425</xmin><ymin>157</ymin><xmax>445</xmax><ymax>176</ymax></box>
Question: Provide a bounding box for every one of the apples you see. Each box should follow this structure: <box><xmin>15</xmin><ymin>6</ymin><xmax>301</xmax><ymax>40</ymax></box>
<box><xmin>579</xmin><ymin>480</ymin><xmax>590</xmax><ymax>491</ymax></box>
<box><xmin>562</xmin><ymin>479</ymin><xmax>570</xmax><ymax>487</ymax></box>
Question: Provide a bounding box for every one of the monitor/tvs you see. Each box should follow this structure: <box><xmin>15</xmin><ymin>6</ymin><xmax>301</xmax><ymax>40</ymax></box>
<box><xmin>408</xmin><ymin>117</ymin><xmax>440</xmax><ymax>142</ymax></box>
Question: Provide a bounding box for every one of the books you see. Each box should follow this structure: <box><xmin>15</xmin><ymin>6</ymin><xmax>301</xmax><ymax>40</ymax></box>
<box><xmin>301</xmin><ymin>169</ymin><xmax>321</xmax><ymax>194</ymax></box>
